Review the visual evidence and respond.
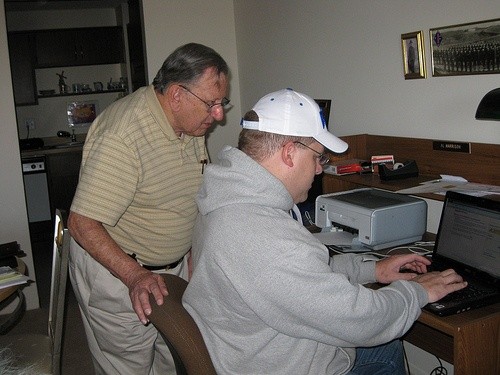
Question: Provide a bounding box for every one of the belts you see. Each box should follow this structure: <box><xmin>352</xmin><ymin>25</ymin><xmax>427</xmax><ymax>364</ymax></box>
<box><xmin>141</xmin><ymin>255</ymin><xmax>185</xmax><ymax>271</ymax></box>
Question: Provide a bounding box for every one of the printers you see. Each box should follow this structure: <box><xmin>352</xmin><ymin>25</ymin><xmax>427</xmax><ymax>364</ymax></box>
<box><xmin>310</xmin><ymin>186</ymin><xmax>428</xmax><ymax>252</ymax></box>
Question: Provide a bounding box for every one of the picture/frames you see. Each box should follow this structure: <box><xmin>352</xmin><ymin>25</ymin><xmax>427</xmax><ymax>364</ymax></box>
<box><xmin>401</xmin><ymin>31</ymin><xmax>427</xmax><ymax>80</ymax></box>
<box><xmin>65</xmin><ymin>100</ymin><xmax>99</xmax><ymax>128</ymax></box>
<box><xmin>429</xmin><ymin>18</ymin><xmax>500</xmax><ymax>78</ymax></box>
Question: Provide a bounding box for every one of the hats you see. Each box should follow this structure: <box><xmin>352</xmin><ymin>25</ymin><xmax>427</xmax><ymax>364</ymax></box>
<box><xmin>239</xmin><ymin>87</ymin><xmax>348</xmax><ymax>153</ymax></box>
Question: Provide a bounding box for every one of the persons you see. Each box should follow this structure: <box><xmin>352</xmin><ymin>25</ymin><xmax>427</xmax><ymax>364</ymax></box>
<box><xmin>68</xmin><ymin>43</ymin><xmax>231</xmax><ymax>375</ymax></box>
<box><xmin>182</xmin><ymin>88</ymin><xmax>468</xmax><ymax>375</ymax></box>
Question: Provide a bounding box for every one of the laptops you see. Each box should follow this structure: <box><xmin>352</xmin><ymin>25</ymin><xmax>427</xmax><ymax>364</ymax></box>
<box><xmin>400</xmin><ymin>191</ymin><xmax>500</xmax><ymax>317</ymax></box>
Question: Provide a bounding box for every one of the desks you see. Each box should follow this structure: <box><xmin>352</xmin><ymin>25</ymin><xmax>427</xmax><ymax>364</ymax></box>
<box><xmin>0</xmin><ymin>259</ymin><xmax>27</xmax><ymax>330</ymax></box>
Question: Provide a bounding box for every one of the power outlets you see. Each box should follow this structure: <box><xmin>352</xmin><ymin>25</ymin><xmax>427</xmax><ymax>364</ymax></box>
<box><xmin>23</xmin><ymin>119</ymin><xmax>35</xmax><ymax>130</ymax></box>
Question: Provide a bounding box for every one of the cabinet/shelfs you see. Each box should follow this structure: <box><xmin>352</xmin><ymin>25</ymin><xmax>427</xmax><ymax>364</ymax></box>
<box><xmin>47</xmin><ymin>150</ymin><xmax>82</xmax><ymax>218</ymax></box>
<box><xmin>7</xmin><ymin>26</ymin><xmax>128</xmax><ymax>105</ymax></box>
<box><xmin>303</xmin><ymin>133</ymin><xmax>500</xmax><ymax>375</ymax></box>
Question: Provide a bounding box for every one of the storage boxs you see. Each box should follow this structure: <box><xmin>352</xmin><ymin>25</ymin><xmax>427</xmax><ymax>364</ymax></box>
<box><xmin>323</xmin><ymin>157</ymin><xmax>368</xmax><ymax>176</ymax></box>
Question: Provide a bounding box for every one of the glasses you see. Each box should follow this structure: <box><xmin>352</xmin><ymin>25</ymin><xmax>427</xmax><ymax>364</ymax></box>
<box><xmin>283</xmin><ymin>141</ymin><xmax>331</xmax><ymax>165</ymax></box>
<box><xmin>178</xmin><ymin>84</ymin><xmax>231</xmax><ymax>113</ymax></box>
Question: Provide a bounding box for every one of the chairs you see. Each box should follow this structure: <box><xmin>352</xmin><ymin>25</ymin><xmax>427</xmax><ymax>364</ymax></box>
<box><xmin>0</xmin><ymin>209</ymin><xmax>70</xmax><ymax>375</ymax></box>
<box><xmin>147</xmin><ymin>274</ymin><xmax>216</xmax><ymax>375</ymax></box>
